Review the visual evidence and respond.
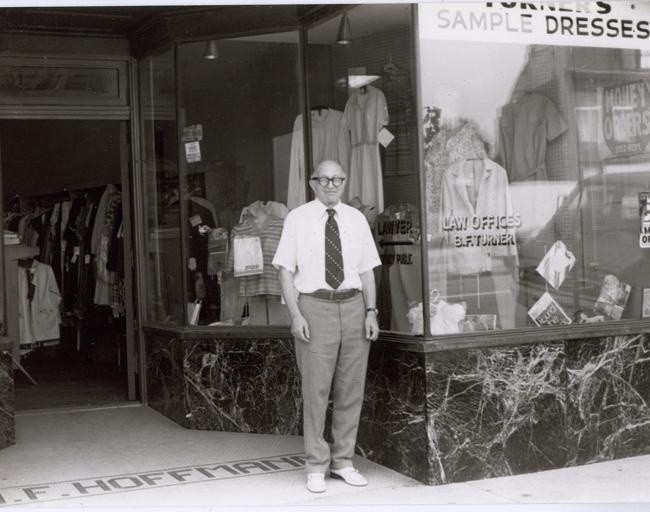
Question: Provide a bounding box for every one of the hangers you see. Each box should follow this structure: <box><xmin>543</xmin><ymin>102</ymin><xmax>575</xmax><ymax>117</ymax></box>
<box><xmin>381</xmin><ymin>56</ymin><xmax>402</xmax><ymax>72</ymax></box>
<box><xmin>308</xmin><ymin>100</ymin><xmax>332</xmax><ymax>113</ymax></box>
<box><xmin>353</xmin><ymin>78</ymin><xmax>378</xmax><ymax>95</ymax></box>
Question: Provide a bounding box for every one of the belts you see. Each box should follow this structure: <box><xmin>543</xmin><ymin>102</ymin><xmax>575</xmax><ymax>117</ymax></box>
<box><xmin>298</xmin><ymin>288</ymin><xmax>364</xmax><ymax>300</ymax></box>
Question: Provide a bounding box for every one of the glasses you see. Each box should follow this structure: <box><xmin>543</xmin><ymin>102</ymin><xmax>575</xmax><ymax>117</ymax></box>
<box><xmin>310</xmin><ymin>177</ymin><xmax>346</xmax><ymax>186</ymax></box>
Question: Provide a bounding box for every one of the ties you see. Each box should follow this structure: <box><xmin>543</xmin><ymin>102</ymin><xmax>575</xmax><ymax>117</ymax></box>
<box><xmin>324</xmin><ymin>208</ymin><xmax>345</xmax><ymax>291</ymax></box>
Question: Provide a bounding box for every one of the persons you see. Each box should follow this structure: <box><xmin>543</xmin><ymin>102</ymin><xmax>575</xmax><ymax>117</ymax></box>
<box><xmin>266</xmin><ymin>158</ymin><xmax>386</xmax><ymax>493</ymax></box>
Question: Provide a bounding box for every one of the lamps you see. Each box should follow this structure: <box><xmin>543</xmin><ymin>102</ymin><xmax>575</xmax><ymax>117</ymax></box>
<box><xmin>203</xmin><ymin>39</ymin><xmax>218</xmax><ymax>58</ymax></box>
<box><xmin>336</xmin><ymin>13</ymin><xmax>350</xmax><ymax>46</ymax></box>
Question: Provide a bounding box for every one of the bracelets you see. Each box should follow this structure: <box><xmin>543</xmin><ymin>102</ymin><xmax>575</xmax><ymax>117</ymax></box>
<box><xmin>365</xmin><ymin>307</ymin><xmax>379</xmax><ymax>314</ymax></box>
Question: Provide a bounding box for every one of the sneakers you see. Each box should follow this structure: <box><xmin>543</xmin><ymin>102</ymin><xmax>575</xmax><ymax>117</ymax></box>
<box><xmin>305</xmin><ymin>472</ymin><xmax>327</xmax><ymax>493</ymax></box>
<box><xmin>330</xmin><ymin>465</ymin><xmax>369</xmax><ymax>486</ymax></box>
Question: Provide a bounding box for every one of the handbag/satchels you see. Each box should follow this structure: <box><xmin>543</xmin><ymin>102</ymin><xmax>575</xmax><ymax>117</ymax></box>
<box><xmin>185</xmin><ymin>299</ymin><xmax>204</xmax><ymax>326</ymax></box>
